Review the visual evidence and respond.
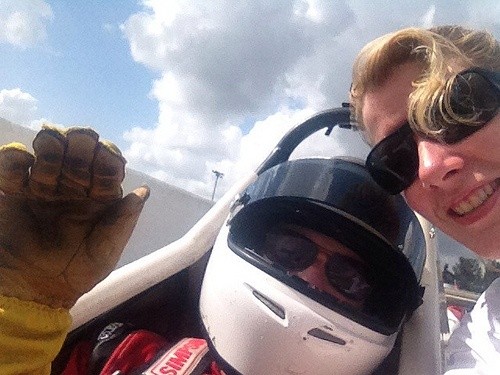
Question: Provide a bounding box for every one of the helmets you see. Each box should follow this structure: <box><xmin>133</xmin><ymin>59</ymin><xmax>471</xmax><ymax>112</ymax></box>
<box><xmin>198</xmin><ymin>155</ymin><xmax>427</xmax><ymax>374</ymax></box>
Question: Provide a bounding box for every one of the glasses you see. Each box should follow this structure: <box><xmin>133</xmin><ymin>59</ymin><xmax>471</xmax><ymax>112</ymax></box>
<box><xmin>262</xmin><ymin>222</ymin><xmax>373</xmax><ymax>302</ymax></box>
<box><xmin>366</xmin><ymin>68</ymin><xmax>500</xmax><ymax>197</ymax></box>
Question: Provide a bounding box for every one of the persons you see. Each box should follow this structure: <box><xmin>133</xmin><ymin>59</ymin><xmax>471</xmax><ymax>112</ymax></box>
<box><xmin>349</xmin><ymin>23</ymin><xmax>500</xmax><ymax>375</ymax></box>
<box><xmin>0</xmin><ymin>124</ymin><xmax>428</xmax><ymax>375</ymax></box>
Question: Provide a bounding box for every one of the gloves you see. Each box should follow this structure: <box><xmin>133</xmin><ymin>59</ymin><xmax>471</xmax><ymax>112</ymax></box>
<box><xmin>0</xmin><ymin>123</ymin><xmax>150</xmax><ymax>309</ymax></box>
